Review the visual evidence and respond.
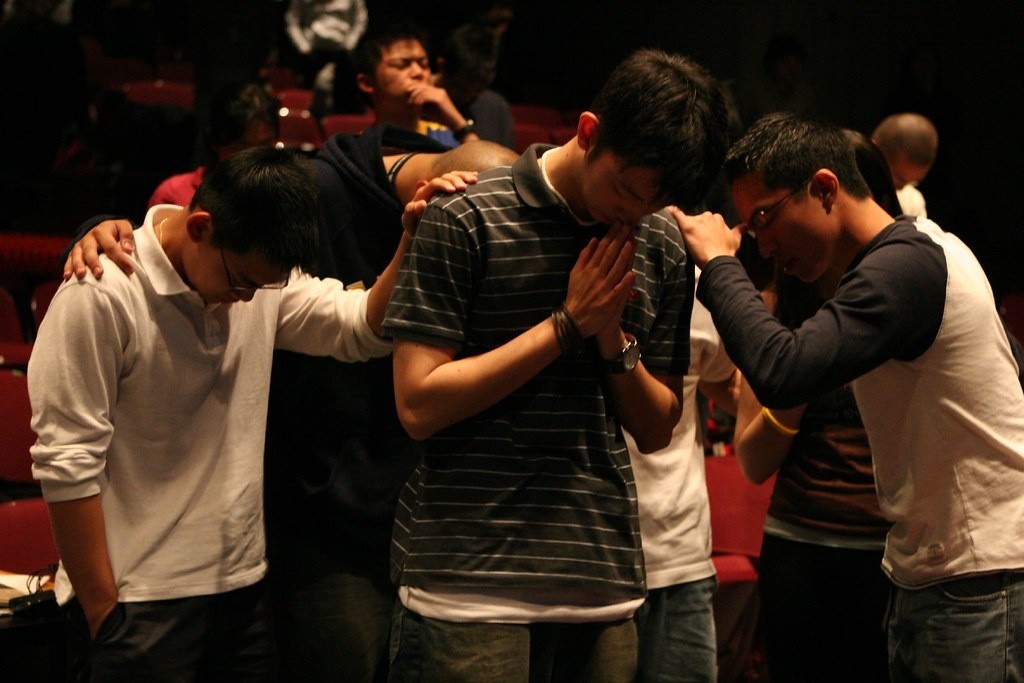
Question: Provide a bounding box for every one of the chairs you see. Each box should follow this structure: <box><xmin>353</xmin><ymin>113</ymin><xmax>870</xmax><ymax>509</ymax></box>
<box><xmin>0</xmin><ymin>68</ymin><xmax>781</xmax><ymax>683</ymax></box>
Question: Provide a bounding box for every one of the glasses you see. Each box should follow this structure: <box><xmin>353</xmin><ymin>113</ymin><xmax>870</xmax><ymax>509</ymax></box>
<box><xmin>219</xmin><ymin>228</ymin><xmax>291</xmax><ymax>291</ymax></box>
<box><xmin>746</xmin><ymin>179</ymin><xmax>812</xmax><ymax>239</ymax></box>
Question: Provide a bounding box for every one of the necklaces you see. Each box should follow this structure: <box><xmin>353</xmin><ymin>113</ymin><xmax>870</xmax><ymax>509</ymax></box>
<box><xmin>160</xmin><ymin>218</ymin><xmax>168</xmax><ymax>248</ymax></box>
<box><xmin>386</xmin><ymin>151</ymin><xmax>414</xmax><ymax>184</ymax></box>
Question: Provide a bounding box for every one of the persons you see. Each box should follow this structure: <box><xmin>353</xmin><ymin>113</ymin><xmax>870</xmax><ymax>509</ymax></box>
<box><xmin>350</xmin><ymin>24</ymin><xmax>480</xmax><ymax>155</ymax></box>
<box><xmin>620</xmin><ymin>265</ymin><xmax>743</xmax><ymax>681</ymax></box>
<box><xmin>381</xmin><ymin>51</ymin><xmax>731</xmax><ymax>682</ymax></box>
<box><xmin>870</xmin><ymin>114</ymin><xmax>938</xmax><ymax>192</ymax></box>
<box><xmin>64</xmin><ymin>141</ymin><xmax>520</xmax><ymax>682</ymax></box>
<box><xmin>758</xmin><ymin>34</ymin><xmax>819</xmax><ymax>120</ymax></box>
<box><xmin>487</xmin><ymin>8</ymin><xmax>511</xmax><ymax>60</ymax></box>
<box><xmin>733</xmin><ymin>130</ymin><xmax>1024</xmax><ymax>683</ymax></box>
<box><xmin>25</xmin><ymin>146</ymin><xmax>477</xmax><ymax>682</ymax></box>
<box><xmin>0</xmin><ymin>0</ymin><xmax>98</xmax><ymax>342</ymax></box>
<box><xmin>881</xmin><ymin>44</ymin><xmax>960</xmax><ymax>232</ymax></box>
<box><xmin>444</xmin><ymin>25</ymin><xmax>493</xmax><ymax>105</ymax></box>
<box><xmin>662</xmin><ymin>113</ymin><xmax>1024</xmax><ymax>682</ymax></box>
<box><xmin>97</xmin><ymin>48</ymin><xmax>198</xmax><ymax>226</ymax></box>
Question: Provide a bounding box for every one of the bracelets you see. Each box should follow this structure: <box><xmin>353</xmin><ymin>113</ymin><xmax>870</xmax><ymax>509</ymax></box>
<box><xmin>553</xmin><ymin>303</ymin><xmax>584</xmax><ymax>357</ymax></box>
<box><xmin>761</xmin><ymin>406</ymin><xmax>798</xmax><ymax>436</ymax></box>
<box><xmin>454</xmin><ymin>119</ymin><xmax>474</xmax><ymax>141</ymax></box>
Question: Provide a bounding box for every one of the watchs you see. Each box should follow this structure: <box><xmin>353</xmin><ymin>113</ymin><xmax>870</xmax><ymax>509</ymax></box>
<box><xmin>602</xmin><ymin>335</ymin><xmax>641</xmax><ymax>375</ymax></box>
